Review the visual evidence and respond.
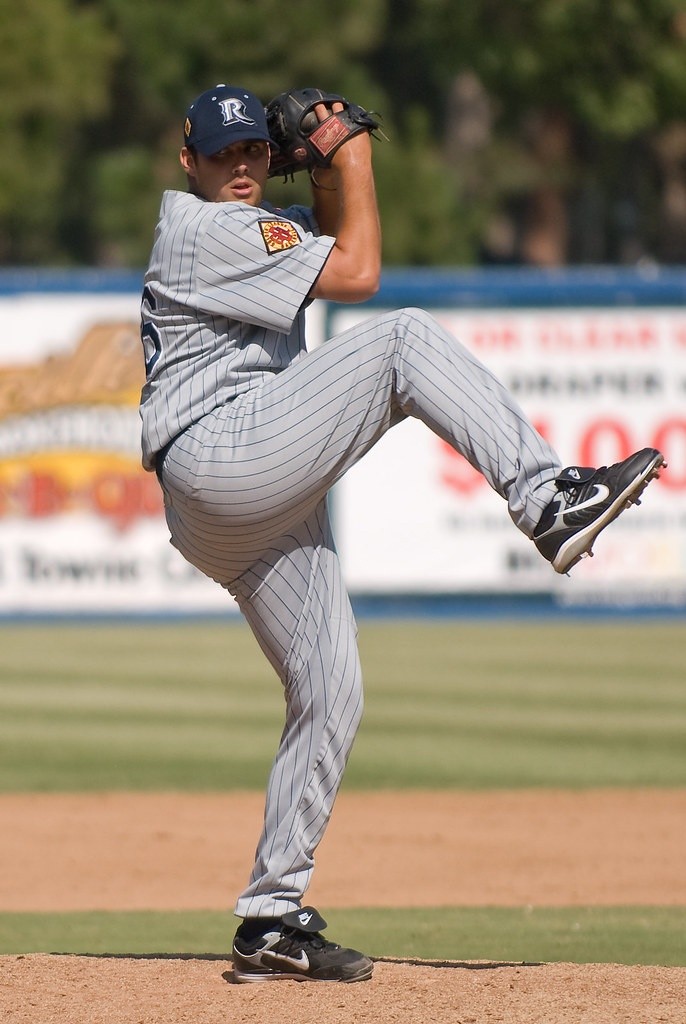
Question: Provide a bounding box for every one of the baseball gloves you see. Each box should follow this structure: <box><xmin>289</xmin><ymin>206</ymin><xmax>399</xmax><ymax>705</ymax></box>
<box><xmin>266</xmin><ymin>71</ymin><xmax>380</xmax><ymax>182</ymax></box>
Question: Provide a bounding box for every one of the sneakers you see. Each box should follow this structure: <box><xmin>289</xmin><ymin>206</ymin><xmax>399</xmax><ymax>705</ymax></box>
<box><xmin>232</xmin><ymin>905</ymin><xmax>382</xmax><ymax>986</ymax></box>
<box><xmin>530</xmin><ymin>445</ymin><xmax>669</xmax><ymax>576</ymax></box>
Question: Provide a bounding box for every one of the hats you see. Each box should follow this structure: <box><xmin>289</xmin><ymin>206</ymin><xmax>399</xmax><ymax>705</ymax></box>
<box><xmin>183</xmin><ymin>84</ymin><xmax>274</xmax><ymax>159</ymax></box>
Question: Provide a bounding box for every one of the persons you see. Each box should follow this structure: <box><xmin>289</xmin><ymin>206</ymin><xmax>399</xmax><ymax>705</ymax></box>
<box><xmin>138</xmin><ymin>81</ymin><xmax>668</xmax><ymax>984</ymax></box>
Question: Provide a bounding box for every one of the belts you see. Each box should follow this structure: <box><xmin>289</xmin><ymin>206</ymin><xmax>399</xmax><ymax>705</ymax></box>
<box><xmin>156</xmin><ymin>414</ymin><xmax>205</xmax><ymax>478</ymax></box>
